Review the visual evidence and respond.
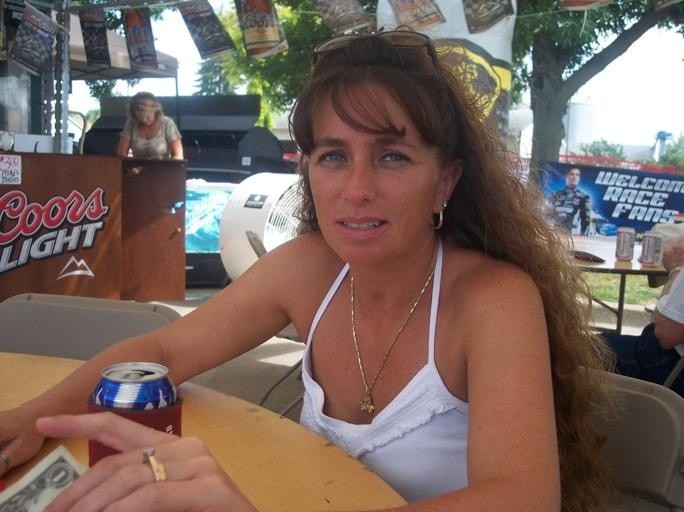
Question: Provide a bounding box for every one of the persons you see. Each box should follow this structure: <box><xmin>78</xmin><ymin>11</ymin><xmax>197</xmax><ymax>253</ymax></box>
<box><xmin>544</xmin><ymin>168</ymin><xmax>591</xmax><ymax>238</ymax></box>
<box><xmin>0</xmin><ymin>23</ymin><xmax>612</xmax><ymax>512</ymax></box>
<box><xmin>115</xmin><ymin>89</ymin><xmax>184</xmax><ymax>161</ymax></box>
<box><xmin>582</xmin><ymin>237</ymin><xmax>684</xmax><ymax>398</ymax></box>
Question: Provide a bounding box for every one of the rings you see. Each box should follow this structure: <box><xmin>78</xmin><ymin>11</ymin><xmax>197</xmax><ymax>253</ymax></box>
<box><xmin>141</xmin><ymin>445</ymin><xmax>165</xmax><ymax>480</ymax></box>
<box><xmin>0</xmin><ymin>451</ymin><xmax>13</xmax><ymax>472</ymax></box>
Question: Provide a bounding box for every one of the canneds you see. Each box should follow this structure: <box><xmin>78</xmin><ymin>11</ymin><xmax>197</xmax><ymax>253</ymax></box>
<box><xmin>89</xmin><ymin>361</ymin><xmax>178</xmax><ymax>411</ymax></box>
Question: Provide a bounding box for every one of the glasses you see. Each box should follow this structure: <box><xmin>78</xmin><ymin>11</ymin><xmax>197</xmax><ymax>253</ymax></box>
<box><xmin>310</xmin><ymin>28</ymin><xmax>445</xmax><ymax>81</ymax></box>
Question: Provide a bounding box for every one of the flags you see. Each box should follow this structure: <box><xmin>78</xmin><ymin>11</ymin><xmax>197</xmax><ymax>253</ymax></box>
<box><xmin>387</xmin><ymin>0</ymin><xmax>447</xmax><ymax>32</ymax></box>
<box><xmin>315</xmin><ymin>1</ymin><xmax>375</xmax><ymax>36</ymax></box>
<box><xmin>80</xmin><ymin>8</ymin><xmax>112</xmax><ymax>65</ymax></box>
<box><xmin>9</xmin><ymin>5</ymin><xmax>58</xmax><ymax>77</ymax></box>
<box><xmin>559</xmin><ymin>1</ymin><xmax>612</xmax><ymax>12</ymax></box>
<box><xmin>463</xmin><ymin>1</ymin><xmax>514</xmax><ymax>34</ymax></box>
<box><xmin>177</xmin><ymin>1</ymin><xmax>237</xmax><ymax>59</ymax></box>
<box><xmin>119</xmin><ymin>7</ymin><xmax>157</xmax><ymax>71</ymax></box>
<box><xmin>234</xmin><ymin>1</ymin><xmax>288</xmax><ymax>60</ymax></box>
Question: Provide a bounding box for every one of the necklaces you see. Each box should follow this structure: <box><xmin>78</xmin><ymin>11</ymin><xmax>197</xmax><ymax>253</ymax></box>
<box><xmin>349</xmin><ymin>266</ymin><xmax>432</xmax><ymax>412</ymax></box>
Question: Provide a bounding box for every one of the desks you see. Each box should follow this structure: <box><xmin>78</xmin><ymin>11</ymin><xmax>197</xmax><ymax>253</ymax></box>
<box><xmin>2</xmin><ymin>350</ymin><xmax>410</xmax><ymax>512</ymax></box>
<box><xmin>556</xmin><ymin>232</ymin><xmax>670</xmax><ymax>339</ymax></box>
